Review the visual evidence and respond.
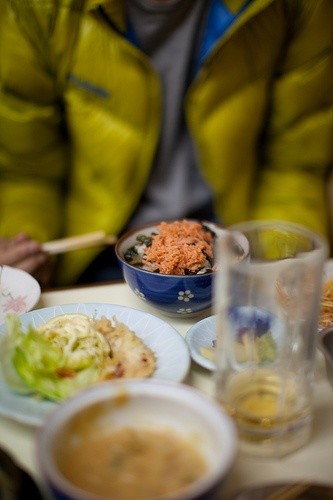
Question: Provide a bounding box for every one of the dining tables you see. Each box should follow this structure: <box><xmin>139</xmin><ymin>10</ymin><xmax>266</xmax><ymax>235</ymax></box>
<box><xmin>0</xmin><ymin>283</ymin><xmax>333</xmax><ymax>500</ymax></box>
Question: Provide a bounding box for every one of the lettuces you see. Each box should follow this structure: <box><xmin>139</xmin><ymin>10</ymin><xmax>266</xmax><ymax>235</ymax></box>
<box><xmin>0</xmin><ymin>312</ymin><xmax>114</xmax><ymax>403</ymax></box>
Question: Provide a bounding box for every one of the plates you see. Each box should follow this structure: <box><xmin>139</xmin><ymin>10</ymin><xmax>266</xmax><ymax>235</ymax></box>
<box><xmin>0</xmin><ymin>265</ymin><xmax>41</xmax><ymax>323</ymax></box>
<box><xmin>186</xmin><ymin>306</ymin><xmax>301</xmax><ymax>373</ymax></box>
<box><xmin>1</xmin><ymin>303</ymin><xmax>190</xmax><ymax>428</ymax></box>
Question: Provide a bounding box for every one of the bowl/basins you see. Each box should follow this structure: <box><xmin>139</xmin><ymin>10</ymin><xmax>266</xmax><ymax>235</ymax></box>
<box><xmin>114</xmin><ymin>220</ymin><xmax>250</xmax><ymax>319</ymax></box>
<box><xmin>34</xmin><ymin>377</ymin><xmax>241</xmax><ymax>500</ymax></box>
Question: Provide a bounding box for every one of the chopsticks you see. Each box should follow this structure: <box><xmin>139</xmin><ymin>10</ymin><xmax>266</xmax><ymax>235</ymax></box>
<box><xmin>39</xmin><ymin>231</ymin><xmax>117</xmax><ymax>255</ymax></box>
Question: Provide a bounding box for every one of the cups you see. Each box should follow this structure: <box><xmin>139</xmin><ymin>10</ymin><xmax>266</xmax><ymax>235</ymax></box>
<box><xmin>213</xmin><ymin>221</ymin><xmax>326</xmax><ymax>455</ymax></box>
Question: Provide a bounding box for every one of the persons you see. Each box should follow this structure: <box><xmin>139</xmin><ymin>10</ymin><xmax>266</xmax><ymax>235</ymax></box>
<box><xmin>0</xmin><ymin>0</ymin><xmax>333</xmax><ymax>294</ymax></box>
